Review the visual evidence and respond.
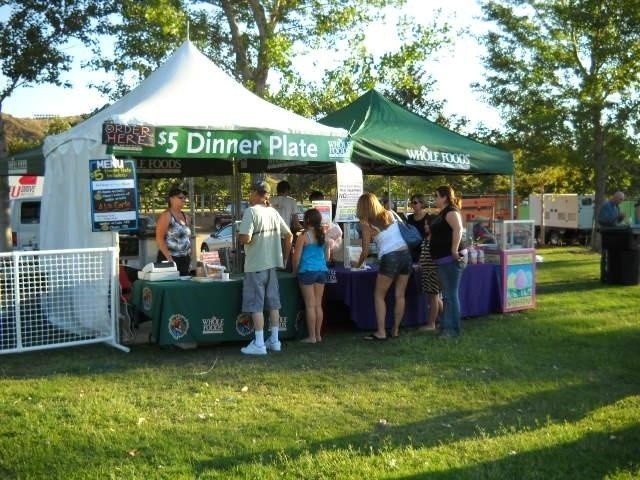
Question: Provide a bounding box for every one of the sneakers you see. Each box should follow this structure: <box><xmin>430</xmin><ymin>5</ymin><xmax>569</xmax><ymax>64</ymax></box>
<box><xmin>300</xmin><ymin>336</ymin><xmax>321</xmax><ymax>344</ymax></box>
<box><xmin>417</xmin><ymin>325</ymin><xmax>452</xmax><ymax>339</ymax></box>
<box><xmin>241</xmin><ymin>338</ymin><xmax>267</xmax><ymax>355</ymax></box>
<box><xmin>265</xmin><ymin>336</ymin><xmax>281</xmax><ymax>351</ymax></box>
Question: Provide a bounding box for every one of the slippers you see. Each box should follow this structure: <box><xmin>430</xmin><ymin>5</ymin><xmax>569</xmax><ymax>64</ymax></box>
<box><xmin>364</xmin><ymin>334</ymin><xmax>387</xmax><ymax>340</ymax></box>
<box><xmin>387</xmin><ymin>331</ymin><xmax>399</xmax><ymax>338</ymax></box>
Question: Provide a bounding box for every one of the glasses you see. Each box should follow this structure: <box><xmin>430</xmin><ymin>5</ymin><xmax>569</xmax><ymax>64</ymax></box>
<box><xmin>410</xmin><ymin>200</ymin><xmax>420</xmax><ymax>204</ymax></box>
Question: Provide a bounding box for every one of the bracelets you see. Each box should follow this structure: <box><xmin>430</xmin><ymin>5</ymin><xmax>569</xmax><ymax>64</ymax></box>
<box><xmin>167</xmin><ymin>256</ymin><xmax>172</xmax><ymax>259</ymax></box>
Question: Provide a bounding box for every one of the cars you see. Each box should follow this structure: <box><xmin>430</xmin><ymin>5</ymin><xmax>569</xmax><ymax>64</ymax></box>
<box><xmin>200</xmin><ymin>220</ymin><xmax>242</xmax><ymax>252</ymax></box>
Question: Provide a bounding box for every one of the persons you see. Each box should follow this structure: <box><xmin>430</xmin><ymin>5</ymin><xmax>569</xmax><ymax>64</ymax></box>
<box><xmin>157</xmin><ymin>188</ymin><xmax>196</xmax><ymax>276</ymax></box>
<box><xmin>269</xmin><ymin>181</ymin><xmax>304</xmax><ymax>251</ymax></box>
<box><xmin>429</xmin><ymin>185</ymin><xmax>469</xmax><ymax>337</ymax></box>
<box><xmin>350</xmin><ymin>193</ymin><xmax>413</xmax><ymax>340</ymax></box>
<box><xmin>418</xmin><ymin>214</ymin><xmax>443</xmax><ymax>332</ymax></box>
<box><xmin>309</xmin><ymin>191</ymin><xmax>344</xmax><ymax>238</ymax></box>
<box><xmin>236</xmin><ymin>181</ymin><xmax>294</xmax><ymax>355</ymax></box>
<box><xmin>408</xmin><ymin>193</ymin><xmax>430</xmax><ymax>263</ymax></box>
<box><xmin>292</xmin><ymin>209</ymin><xmax>330</xmax><ymax>343</ymax></box>
<box><xmin>598</xmin><ymin>190</ymin><xmax>625</xmax><ymax>228</ymax></box>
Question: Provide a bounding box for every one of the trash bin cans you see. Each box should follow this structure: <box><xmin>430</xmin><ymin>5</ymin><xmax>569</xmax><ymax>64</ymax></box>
<box><xmin>595</xmin><ymin>225</ymin><xmax>640</xmax><ymax>286</ymax></box>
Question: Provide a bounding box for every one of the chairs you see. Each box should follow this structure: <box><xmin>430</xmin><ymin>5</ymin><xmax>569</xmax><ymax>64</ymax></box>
<box><xmin>119</xmin><ymin>268</ymin><xmax>135</xmax><ymax>336</ymax></box>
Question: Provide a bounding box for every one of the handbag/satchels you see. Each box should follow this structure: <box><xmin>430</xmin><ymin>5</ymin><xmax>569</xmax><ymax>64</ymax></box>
<box><xmin>399</xmin><ymin>222</ymin><xmax>423</xmax><ymax>249</ymax></box>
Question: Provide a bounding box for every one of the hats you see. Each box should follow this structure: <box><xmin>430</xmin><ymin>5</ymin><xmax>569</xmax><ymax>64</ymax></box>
<box><xmin>169</xmin><ymin>188</ymin><xmax>187</xmax><ymax>197</ymax></box>
<box><xmin>247</xmin><ymin>180</ymin><xmax>271</xmax><ymax>195</ymax></box>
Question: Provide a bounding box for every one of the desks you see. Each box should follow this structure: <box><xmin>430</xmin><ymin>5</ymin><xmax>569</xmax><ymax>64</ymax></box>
<box><xmin>132</xmin><ymin>259</ymin><xmax>499</xmax><ymax>349</ymax></box>
